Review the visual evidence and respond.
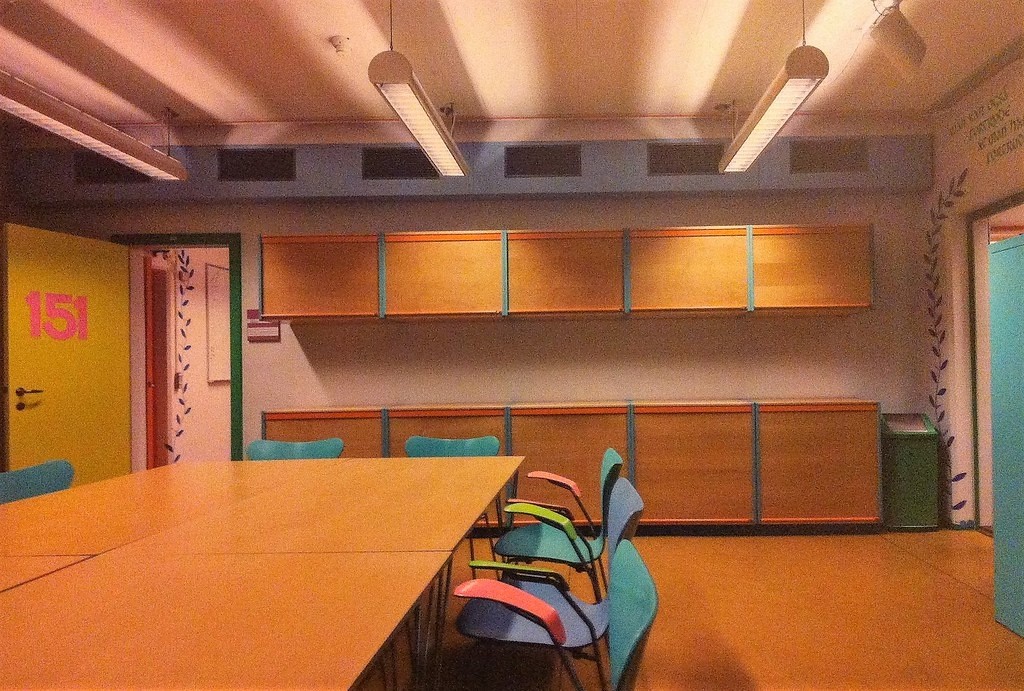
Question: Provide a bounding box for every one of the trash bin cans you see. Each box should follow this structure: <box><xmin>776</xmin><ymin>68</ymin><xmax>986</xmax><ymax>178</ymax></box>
<box><xmin>881</xmin><ymin>412</ymin><xmax>939</xmax><ymax>527</ymax></box>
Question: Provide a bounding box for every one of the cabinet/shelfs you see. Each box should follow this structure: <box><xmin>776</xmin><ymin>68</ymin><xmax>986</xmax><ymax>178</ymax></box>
<box><xmin>261</xmin><ymin>399</ymin><xmax>881</xmax><ymax>525</ymax></box>
<box><xmin>257</xmin><ymin>225</ymin><xmax>873</xmax><ymax>320</ymax></box>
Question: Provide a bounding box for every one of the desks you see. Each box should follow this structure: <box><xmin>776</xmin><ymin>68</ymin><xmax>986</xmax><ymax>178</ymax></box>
<box><xmin>0</xmin><ymin>456</ymin><xmax>527</xmax><ymax>691</ymax></box>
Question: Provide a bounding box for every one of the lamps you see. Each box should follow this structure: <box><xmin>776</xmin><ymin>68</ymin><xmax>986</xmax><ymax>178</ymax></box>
<box><xmin>718</xmin><ymin>46</ymin><xmax>830</xmax><ymax>173</ymax></box>
<box><xmin>1</xmin><ymin>70</ymin><xmax>188</xmax><ymax>181</ymax></box>
<box><xmin>368</xmin><ymin>50</ymin><xmax>469</xmax><ymax>177</ymax></box>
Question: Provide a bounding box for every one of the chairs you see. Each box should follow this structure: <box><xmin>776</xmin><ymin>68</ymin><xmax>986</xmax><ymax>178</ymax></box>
<box><xmin>247</xmin><ymin>438</ymin><xmax>344</xmax><ymax>460</ymax></box>
<box><xmin>454</xmin><ymin>448</ymin><xmax>658</xmax><ymax>690</ymax></box>
<box><xmin>404</xmin><ymin>435</ymin><xmax>501</xmax><ymax>579</ymax></box>
<box><xmin>0</xmin><ymin>460</ymin><xmax>74</xmax><ymax>504</ymax></box>
